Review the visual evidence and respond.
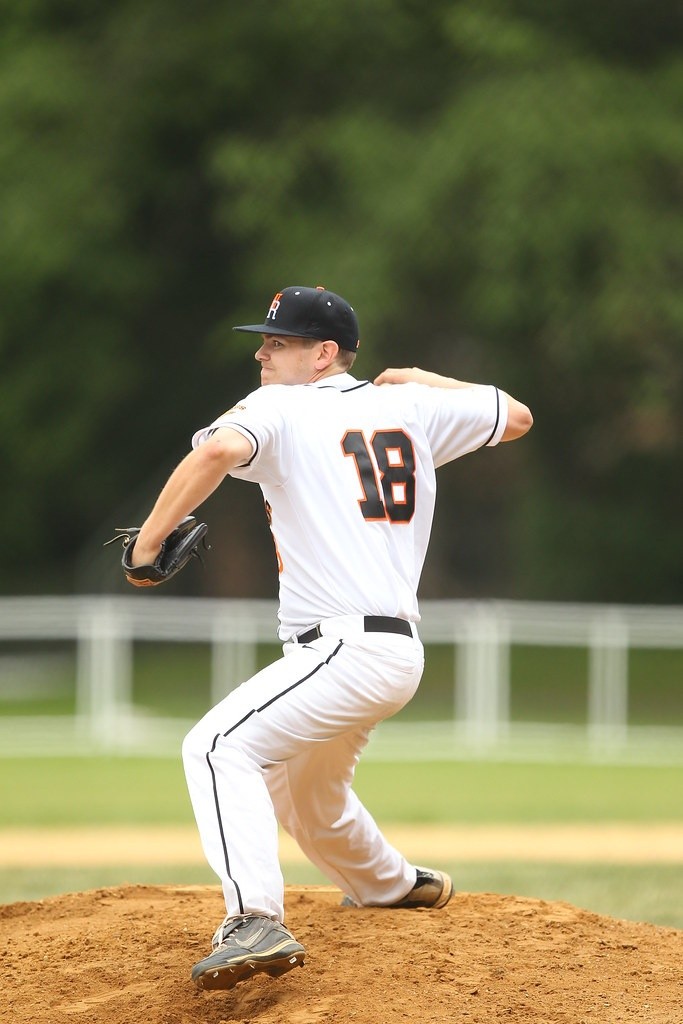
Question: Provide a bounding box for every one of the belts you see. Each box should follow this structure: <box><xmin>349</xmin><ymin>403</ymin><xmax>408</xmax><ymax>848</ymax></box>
<box><xmin>291</xmin><ymin>615</ymin><xmax>413</xmax><ymax>644</ymax></box>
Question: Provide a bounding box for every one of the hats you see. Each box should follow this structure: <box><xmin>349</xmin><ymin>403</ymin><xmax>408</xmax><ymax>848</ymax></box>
<box><xmin>232</xmin><ymin>286</ymin><xmax>360</xmax><ymax>354</ymax></box>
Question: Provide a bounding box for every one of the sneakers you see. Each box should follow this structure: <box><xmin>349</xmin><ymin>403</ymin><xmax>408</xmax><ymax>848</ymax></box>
<box><xmin>339</xmin><ymin>865</ymin><xmax>454</xmax><ymax>909</ymax></box>
<box><xmin>191</xmin><ymin>912</ymin><xmax>306</xmax><ymax>991</ymax></box>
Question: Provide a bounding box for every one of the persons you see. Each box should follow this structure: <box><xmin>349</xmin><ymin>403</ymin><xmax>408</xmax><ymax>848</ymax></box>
<box><xmin>102</xmin><ymin>286</ymin><xmax>534</xmax><ymax>990</ymax></box>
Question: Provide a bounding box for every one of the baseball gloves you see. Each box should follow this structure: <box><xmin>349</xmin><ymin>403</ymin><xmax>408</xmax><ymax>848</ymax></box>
<box><xmin>120</xmin><ymin>514</ymin><xmax>212</xmax><ymax>588</ymax></box>
